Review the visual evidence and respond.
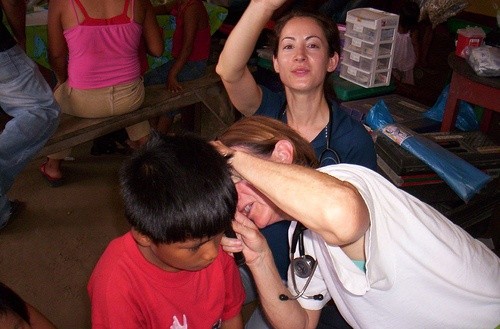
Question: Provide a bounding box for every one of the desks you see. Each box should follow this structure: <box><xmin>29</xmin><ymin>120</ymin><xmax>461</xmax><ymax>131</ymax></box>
<box><xmin>441</xmin><ymin>52</ymin><xmax>500</xmax><ymax>139</ymax></box>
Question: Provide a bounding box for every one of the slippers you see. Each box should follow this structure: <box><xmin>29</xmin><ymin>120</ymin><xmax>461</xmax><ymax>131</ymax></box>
<box><xmin>40</xmin><ymin>160</ymin><xmax>62</xmax><ymax>187</ymax></box>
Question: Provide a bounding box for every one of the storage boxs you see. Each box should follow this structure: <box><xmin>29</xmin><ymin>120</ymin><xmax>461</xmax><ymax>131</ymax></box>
<box><xmin>340</xmin><ymin>8</ymin><xmax>399</xmax><ymax>87</ymax></box>
<box><xmin>339</xmin><ymin>94</ymin><xmax>439</xmax><ymax>139</ymax></box>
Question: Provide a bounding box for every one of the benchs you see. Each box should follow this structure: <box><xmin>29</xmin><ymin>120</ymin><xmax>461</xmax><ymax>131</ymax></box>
<box><xmin>18</xmin><ymin>64</ymin><xmax>256</xmax><ymax>158</ymax></box>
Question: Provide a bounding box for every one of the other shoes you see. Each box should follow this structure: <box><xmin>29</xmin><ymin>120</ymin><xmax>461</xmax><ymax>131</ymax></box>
<box><xmin>0</xmin><ymin>201</ymin><xmax>18</xmax><ymax>230</ymax></box>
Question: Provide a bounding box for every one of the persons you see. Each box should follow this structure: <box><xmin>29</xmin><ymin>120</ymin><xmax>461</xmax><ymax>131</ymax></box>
<box><xmin>38</xmin><ymin>0</ymin><xmax>165</xmax><ymax>180</ymax></box>
<box><xmin>87</xmin><ymin>134</ymin><xmax>245</xmax><ymax>329</ymax></box>
<box><xmin>0</xmin><ymin>0</ymin><xmax>62</xmax><ymax>228</ymax></box>
<box><xmin>127</xmin><ymin>0</ymin><xmax>211</xmax><ymax>152</ymax></box>
<box><xmin>215</xmin><ymin>0</ymin><xmax>377</xmax><ymax>329</ymax></box>
<box><xmin>209</xmin><ymin>115</ymin><xmax>500</xmax><ymax>329</ymax></box>
